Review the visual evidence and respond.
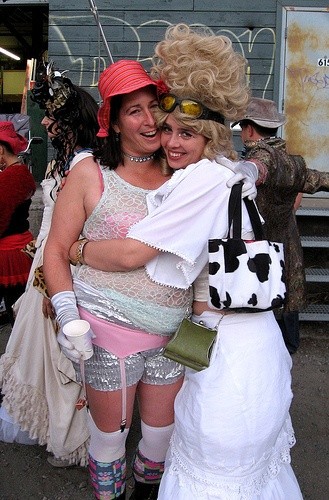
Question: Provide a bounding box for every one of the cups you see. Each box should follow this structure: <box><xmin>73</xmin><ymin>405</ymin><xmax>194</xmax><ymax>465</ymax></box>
<box><xmin>62</xmin><ymin>319</ymin><xmax>94</xmax><ymax>361</ymax></box>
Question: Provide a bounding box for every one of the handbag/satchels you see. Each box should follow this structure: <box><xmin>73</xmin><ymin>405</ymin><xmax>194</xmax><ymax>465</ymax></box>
<box><xmin>161</xmin><ymin>317</ymin><xmax>218</xmax><ymax>373</ymax></box>
<box><xmin>207</xmin><ymin>181</ymin><xmax>288</xmax><ymax>315</ymax></box>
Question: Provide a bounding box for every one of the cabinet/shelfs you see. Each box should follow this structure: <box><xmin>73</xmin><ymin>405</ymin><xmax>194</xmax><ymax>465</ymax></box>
<box><xmin>293</xmin><ymin>206</ymin><xmax>329</xmax><ymax>324</ymax></box>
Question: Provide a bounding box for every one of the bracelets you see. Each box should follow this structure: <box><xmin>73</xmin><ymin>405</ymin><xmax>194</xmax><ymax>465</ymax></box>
<box><xmin>76</xmin><ymin>237</ymin><xmax>90</xmax><ymax>265</ymax></box>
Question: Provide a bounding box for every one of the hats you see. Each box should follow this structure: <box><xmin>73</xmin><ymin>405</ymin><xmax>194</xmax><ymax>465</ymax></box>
<box><xmin>0</xmin><ymin>121</ymin><xmax>29</xmax><ymax>155</ymax></box>
<box><xmin>96</xmin><ymin>58</ymin><xmax>170</xmax><ymax>139</ymax></box>
<box><xmin>231</xmin><ymin>96</ymin><xmax>288</xmax><ymax>128</ymax></box>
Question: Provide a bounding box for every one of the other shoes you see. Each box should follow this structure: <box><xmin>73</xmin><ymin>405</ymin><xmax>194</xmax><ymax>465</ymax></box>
<box><xmin>95</xmin><ymin>471</ymin><xmax>160</xmax><ymax>500</ymax></box>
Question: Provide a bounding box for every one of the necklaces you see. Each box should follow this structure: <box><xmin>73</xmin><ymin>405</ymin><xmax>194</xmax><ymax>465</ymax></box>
<box><xmin>3</xmin><ymin>160</ymin><xmax>21</xmax><ymax>170</ymax></box>
<box><xmin>122</xmin><ymin>149</ymin><xmax>158</xmax><ymax>162</ymax></box>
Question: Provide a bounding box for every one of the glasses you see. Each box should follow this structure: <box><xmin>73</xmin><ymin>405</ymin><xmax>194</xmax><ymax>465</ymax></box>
<box><xmin>158</xmin><ymin>92</ymin><xmax>225</xmax><ymax>126</ymax></box>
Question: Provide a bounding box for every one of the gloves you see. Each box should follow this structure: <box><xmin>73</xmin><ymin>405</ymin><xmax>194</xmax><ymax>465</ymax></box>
<box><xmin>215</xmin><ymin>154</ymin><xmax>258</xmax><ymax>201</ymax></box>
<box><xmin>50</xmin><ymin>291</ymin><xmax>97</xmax><ymax>365</ymax></box>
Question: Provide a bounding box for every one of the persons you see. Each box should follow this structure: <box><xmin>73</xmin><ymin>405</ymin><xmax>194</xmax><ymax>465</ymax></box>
<box><xmin>42</xmin><ymin>62</ymin><xmax>268</xmax><ymax>500</ymax></box>
<box><xmin>43</xmin><ymin>60</ymin><xmax>186</xmax><ymax>500</ymax></box>
<box><xmin>42</xmin><ymin>22</ymin><xmax>305</xmax><ymax>500</ymax></box>
<box><xmin>0</xmin><ymin>57</ymin><xmax>100</xmax><ymax>467</ymax></box>
<box><xmin>228</xmin><ymin>93</ymin><xmax>328</xmax><ymax>353</ymax></box>
<box><xmin>1</xmin><ymin>121</ymin><xmax>40</xmax><ymax>336</ymax></box>
